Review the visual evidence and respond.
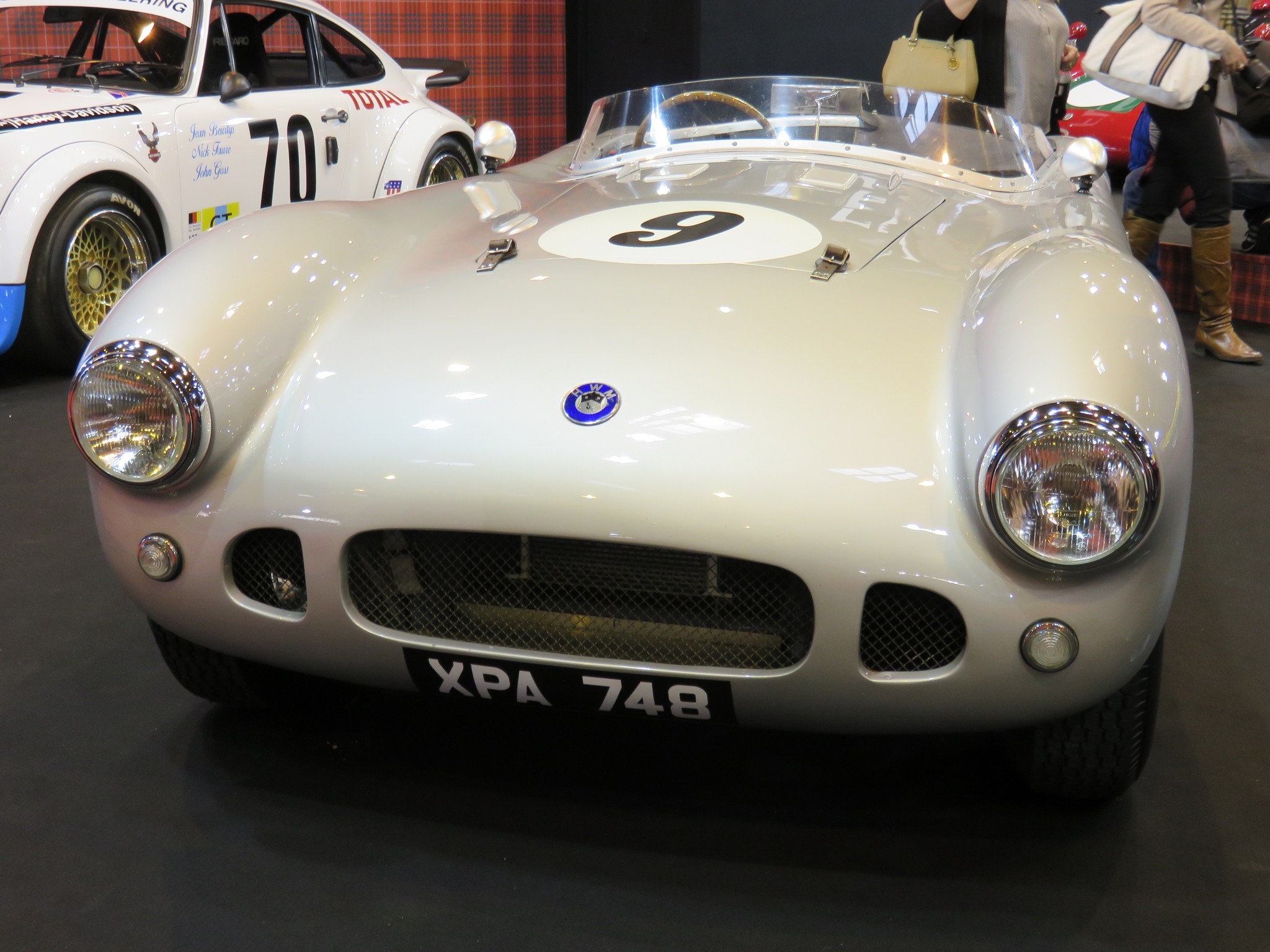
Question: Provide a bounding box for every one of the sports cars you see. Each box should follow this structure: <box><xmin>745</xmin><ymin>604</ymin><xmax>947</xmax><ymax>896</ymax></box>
<box><xmin>0</xmin><ymin>1</ymin><xmax>485</xmax><ymax>376</ymax></box>
<box><xmin>67</xmin><ymin>71</ymin><xmax>1198</xmax><ymax>807</ymax></box>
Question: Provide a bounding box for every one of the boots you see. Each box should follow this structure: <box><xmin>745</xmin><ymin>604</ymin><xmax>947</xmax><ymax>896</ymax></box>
<box><xmin>1189</xmin><ymin>223</ymin><xmax>1262</xmax><ymax>362</ymax></box>
<box><xmin>1122</xmin><ymin>208</ymin><xmax>1163</xmax><ymax>266</ymax></box>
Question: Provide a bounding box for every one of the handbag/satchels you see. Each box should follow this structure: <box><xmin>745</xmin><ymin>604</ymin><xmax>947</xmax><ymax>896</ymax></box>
<box><xmin>882</xmin><ymin>10</ymin><xmax>978</xmax><ymax>104</ymax></box>
<box><xmin>1081</xmin><ymin>0</ymin><xmax>1209</xmax><ymax>110</ymax></box>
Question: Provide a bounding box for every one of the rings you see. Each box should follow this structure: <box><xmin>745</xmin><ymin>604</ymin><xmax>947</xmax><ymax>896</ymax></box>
<box><xmin>1239</xmin><ymin>64</ymin><xmax>1244</xmax><ymax>69</ymax></box>
<box><xmin>1070</xmin><ymin>66</ymin><xmax>1073</xmax><ymax>68</ymax></box>
<box><xmin>1069</xmin><ymin>63</ymin><xmax>1072</xmax><ymax>66</ymax></box>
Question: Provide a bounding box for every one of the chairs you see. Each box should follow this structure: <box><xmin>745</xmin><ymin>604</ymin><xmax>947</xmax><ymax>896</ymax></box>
<box><xmin>201</xmin><ymin>11</ymin><xmax>275</xmax><ymax>90</ymax></box>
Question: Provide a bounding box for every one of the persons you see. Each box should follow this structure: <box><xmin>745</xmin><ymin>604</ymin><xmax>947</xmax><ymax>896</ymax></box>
<box><xmin>1122</xmin><ymin>0</ymin><xmax>1270</xmax><ymax>362</ymax></box>
<box><xmin>914</xmin><ymin>0</ymin><xmax>1080</xmax><ymax>138</ymax></box>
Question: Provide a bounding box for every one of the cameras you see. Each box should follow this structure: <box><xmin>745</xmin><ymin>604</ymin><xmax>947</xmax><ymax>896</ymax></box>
<box><xmin>1238</xmin><ymin>46</ymin><xmax>1270</xmax><ymax>89</ymax></box>
